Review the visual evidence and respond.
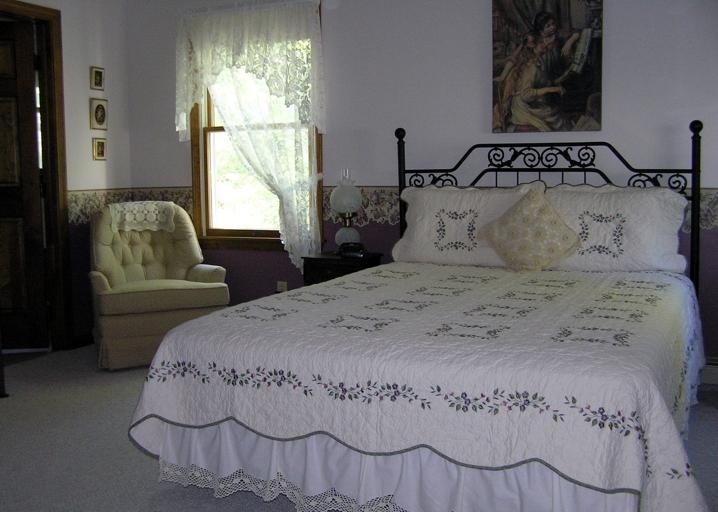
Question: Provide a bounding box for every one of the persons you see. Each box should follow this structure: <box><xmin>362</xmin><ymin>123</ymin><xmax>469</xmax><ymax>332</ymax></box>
<box><xmin>491</xmin><ymin>0</ymin><xmax>602</xmax><ymax>134</ymax></box>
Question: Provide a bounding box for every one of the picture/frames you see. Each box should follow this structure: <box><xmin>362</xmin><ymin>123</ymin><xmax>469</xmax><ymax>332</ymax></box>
<box><xmin>90</xmin><ymin>66</ymin><xmax>106</xmax><ymax>90</ymax></box>
<box><xmin>89</xmin><ymin>97</ymin><xmax>109</xmax><ymax>130</ymax></box>
<box><xmin>93</xmin><ymin>137</ymin><xmax>107</xmax><ymax>160</ymax></box>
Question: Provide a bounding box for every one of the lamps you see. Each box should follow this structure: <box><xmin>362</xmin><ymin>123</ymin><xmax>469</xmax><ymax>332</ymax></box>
<box><xmin>328</xmin><ymin>162</ymin><xmax>367</xmax><ymax>253</ymax></box>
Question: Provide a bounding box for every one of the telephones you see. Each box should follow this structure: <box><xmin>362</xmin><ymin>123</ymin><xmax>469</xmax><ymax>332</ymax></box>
<box><xmin>338</xmin><ymin>242</ymin><xmax>368</xmax><ymax>259</ymax></box>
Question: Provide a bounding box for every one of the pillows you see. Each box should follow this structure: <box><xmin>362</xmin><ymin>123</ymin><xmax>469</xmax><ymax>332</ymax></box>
<box><xmin>546</xmin><ymin>182</ymin><xmax>687</xmax><ymax>273</ymax></box>
<box><xmin>477</xmin><ymin>185</ymin><xmax>581</xmax><ymax>274</ymax></box>
<box><xmin>397</xmin><ymin>181</ymin><xmax>543</xmax><ymax>266</ymax></box>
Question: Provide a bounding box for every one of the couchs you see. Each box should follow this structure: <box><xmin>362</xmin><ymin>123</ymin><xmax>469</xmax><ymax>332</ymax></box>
<box><xmin>82</xmin><ymin>199</ymin><xmax>233</xmax><ymax>371</ymax></box>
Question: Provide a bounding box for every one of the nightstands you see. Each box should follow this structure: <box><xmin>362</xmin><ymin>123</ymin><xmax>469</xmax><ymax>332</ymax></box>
<box><xmin>299</xmin><ymin>248</ymin><xmax>391</xmax><ymax>284</ymax></box>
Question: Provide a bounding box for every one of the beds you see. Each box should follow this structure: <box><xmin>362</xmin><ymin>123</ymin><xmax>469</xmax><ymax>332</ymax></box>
<box><xmin>124</xmin><ymin>118</ymin><xmax>708</xmax><ymax>512</ymax></box>
